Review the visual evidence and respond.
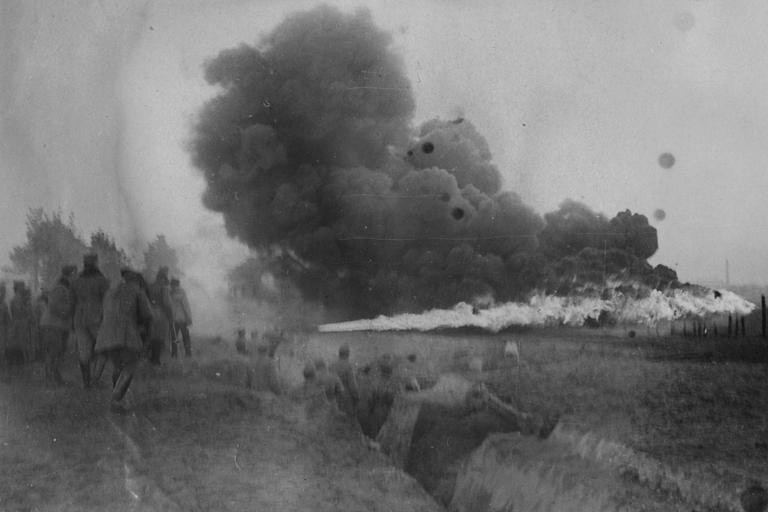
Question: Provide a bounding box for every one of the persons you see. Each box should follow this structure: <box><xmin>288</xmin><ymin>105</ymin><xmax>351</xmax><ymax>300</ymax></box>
<box><xmin>228</xmin><ymin>326</ymin><xmax>421</xmax><ymax>417</ymax></box>
<box><xmin>1</xmin><ymin>252</ymin><xmax>195</xmax><ymax>414</ymax></box>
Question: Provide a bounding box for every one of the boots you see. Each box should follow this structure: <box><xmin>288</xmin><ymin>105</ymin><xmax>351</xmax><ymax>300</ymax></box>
<box><xmin>77</xmin><ymin>329</ymin><xmax>192</xmax><ymax>414</ymax></box>
